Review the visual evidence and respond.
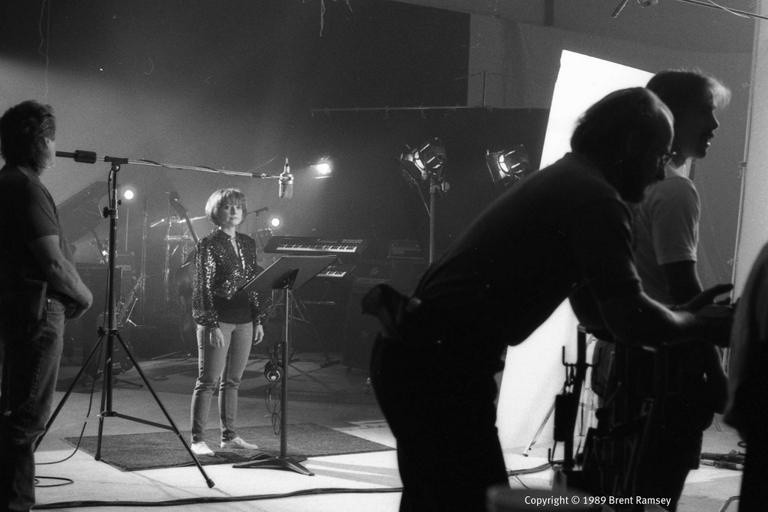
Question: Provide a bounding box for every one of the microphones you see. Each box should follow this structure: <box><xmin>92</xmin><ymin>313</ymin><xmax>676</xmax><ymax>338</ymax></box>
<box><xmin>255</xmin><ymin>206</ymin><xmax>268</xmax><ymax>215</ymax></box>
<box><xmin>277</xmin><ymin>157</ymin><xmax>294</xmax><ymax>199</ymax></box>
<box><xmin>168</xmin><ymin>192</ymin><xmax>189</xmax><ymax>221</ymax></box>
<box><xmin>149</xmin><ymin>215</ymin><xmax>167</xmax><ymax>227</ymax></box>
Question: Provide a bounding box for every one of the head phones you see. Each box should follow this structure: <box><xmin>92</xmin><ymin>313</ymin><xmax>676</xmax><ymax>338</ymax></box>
<box><xmin>263</xmin><ymin>340</ymin><xmax>285</xmax><ymax>384</ymax></box>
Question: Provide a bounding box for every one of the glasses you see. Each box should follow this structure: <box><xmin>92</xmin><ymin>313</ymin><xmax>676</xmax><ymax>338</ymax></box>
<box><xmin>657</xmin><ymin>149</ymin><xmax>678</xmax><ymax>170</ymax></box>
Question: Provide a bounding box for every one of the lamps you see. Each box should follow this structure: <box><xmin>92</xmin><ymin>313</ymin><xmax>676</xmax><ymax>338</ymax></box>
<box><xmin>388</xmin><ymin>141</ymin><xmax>444</xmax><ymax>268</ymax></box>
<box><xmin>477</xmin><ymin>139</ymin><xmax>530</xmax><ymax>199</ymax></box>
<box><xmin>120</xmin><ymin>184</ymin><xmax>140</xmax><ymax>250</ymax></box>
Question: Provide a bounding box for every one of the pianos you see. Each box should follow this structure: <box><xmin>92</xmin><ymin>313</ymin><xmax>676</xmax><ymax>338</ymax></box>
<box><xmin>263</xmin><ymin>235</ymin><xmax>364</xmax><ymax>257</ymax></box>
<box><xmin>308</xmin><ymin>261</ymin><xmax>369</xmax><ymax>286</ymax></box>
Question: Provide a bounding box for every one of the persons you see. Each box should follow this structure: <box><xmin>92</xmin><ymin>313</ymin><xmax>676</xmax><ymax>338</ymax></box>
<box><xmin>724</xmin><ymin>242</ymin><xmax>767</xmax><ymax>512</ymax></box>
<box><xmin>362</xmin><ymin>87</ymin><xmax>736</xmax><ymax>512</ymax></box>
<box><xmin>593</xmin><ymin>70</ymin><xmax>730</xmax><ymax>512</ymax></box>
<box><xmin>191</xmin><ymin>188</ymin><xmax>264</xmax><ymax>455</ymax></box>
<box><xmin>0</xmin><ymin>100</ymin><xmax>94</xmax><ymax>512</ymax></box>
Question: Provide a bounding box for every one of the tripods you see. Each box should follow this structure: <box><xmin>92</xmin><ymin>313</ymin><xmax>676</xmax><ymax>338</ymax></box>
<box><xmin>34</xmin><ymin>222</ymin><xmax>215</xmax><ymax>491</ymax></box>
<box><xmin>232</xmin><ymin>290</ymin><xmax>316</xmax><ymax>477</ymax></box>
<box><xmin>521</xmin><ymin>337</ymin><xmax>599</xmax><ymax>459</ymax></box>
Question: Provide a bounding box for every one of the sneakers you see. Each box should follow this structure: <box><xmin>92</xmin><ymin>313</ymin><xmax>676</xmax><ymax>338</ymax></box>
<box><xmin>221</xmin><ymin>436</ymin><xmax>259</xmax><ymax>450</ymax></box>
<box><xmin>191</xmin><ymin>441</ymin><xmax>215</xmax><ymax>456</ymax></box>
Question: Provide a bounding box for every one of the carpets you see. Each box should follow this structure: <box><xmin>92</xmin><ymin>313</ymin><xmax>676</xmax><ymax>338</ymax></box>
<box><xmin>62</xmin><ymin>421</ymin><xmax>396</xmax><ymax>472</ymax></box>
